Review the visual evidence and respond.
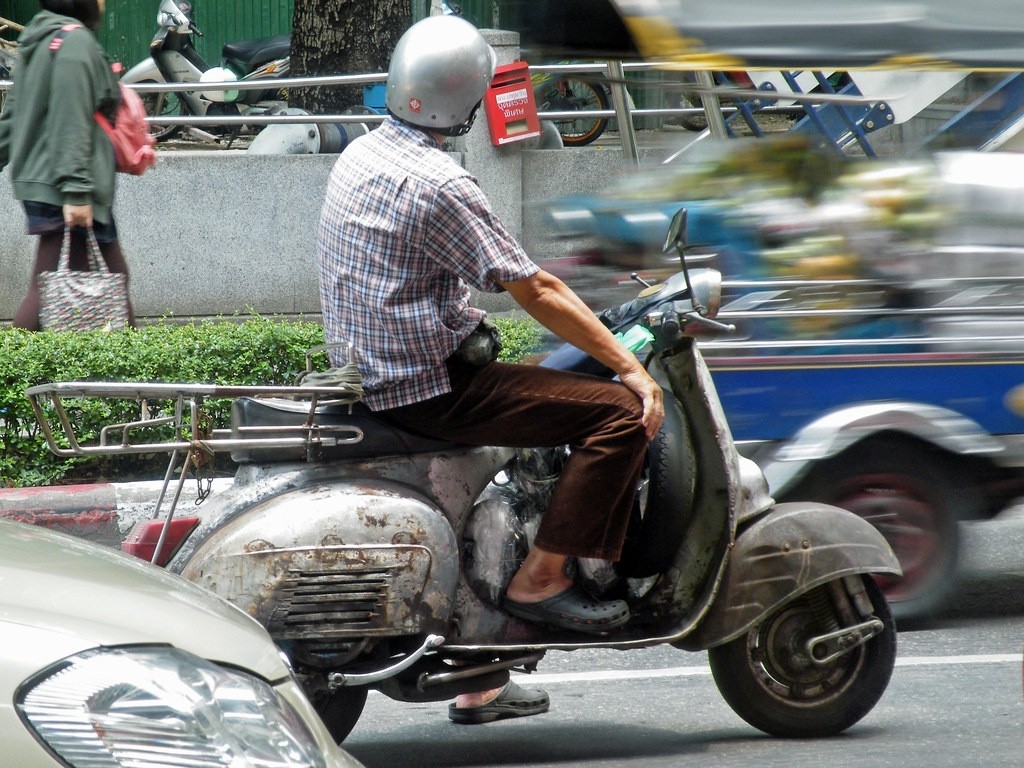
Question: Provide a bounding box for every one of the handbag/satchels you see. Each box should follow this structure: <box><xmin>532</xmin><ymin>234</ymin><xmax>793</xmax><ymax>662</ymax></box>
<box><xmin>37</xmin><ymin>225</ymin><xmax>130</xmax><ymax>332</ymax></box>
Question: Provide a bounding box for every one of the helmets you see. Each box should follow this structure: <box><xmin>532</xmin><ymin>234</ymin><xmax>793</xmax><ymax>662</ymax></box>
<box><xmin>387</xmin><ymin>14</ymin><xmax>498</xmax><ymax>137</ymax></box>
<box><xmin>199</xmin><ymin>67</ymin><xmax>239</xmax><ymax>102</ymax></box>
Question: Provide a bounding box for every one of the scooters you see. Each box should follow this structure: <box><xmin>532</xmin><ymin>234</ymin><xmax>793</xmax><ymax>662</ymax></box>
<box><xmin>25</xmin><ymin>205</ymin><xmax>902</xmax><ymax>746</ymax></box>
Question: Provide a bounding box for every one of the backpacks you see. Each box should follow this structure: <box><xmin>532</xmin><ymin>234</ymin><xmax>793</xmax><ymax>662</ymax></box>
<box><xmin>49</xmin><ymin>22</ymin><xmax>156</xmax><ymax>176</ymax></box>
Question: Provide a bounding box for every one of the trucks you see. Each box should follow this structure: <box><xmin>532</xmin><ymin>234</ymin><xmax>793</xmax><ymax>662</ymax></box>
<box><xmin>532</xmin><ymin>191</ymin><xmax>1024</xmax><ymax>629</ymax></box>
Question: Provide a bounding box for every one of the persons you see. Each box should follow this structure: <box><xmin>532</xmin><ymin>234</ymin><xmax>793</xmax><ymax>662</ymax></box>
<box><xmin>319</xmin><ymin>16</ymin><xmax>666</xmax><ymax>724</ymax></box>
<box><xmin>0</xmin><ymin>0</ymin><xmax>136</xmax><ymax>332</ymax></box>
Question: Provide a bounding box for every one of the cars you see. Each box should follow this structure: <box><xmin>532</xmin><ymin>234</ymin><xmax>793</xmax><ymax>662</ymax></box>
<box><xmin>0</xmin><ymin>517</ymin><xmax>372</xmax><ymax>768</ymax></box>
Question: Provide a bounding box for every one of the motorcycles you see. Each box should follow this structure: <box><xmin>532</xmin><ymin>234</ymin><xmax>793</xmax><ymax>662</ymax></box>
<box><xmin>117</xmin><ymin>0</ymin><xmax>292</xmax><ymax>148</ymax></box>
<box><xmin>428</xmin><ymin>0</ymin><xmax>614</xmax><ymax>147</ymax></box>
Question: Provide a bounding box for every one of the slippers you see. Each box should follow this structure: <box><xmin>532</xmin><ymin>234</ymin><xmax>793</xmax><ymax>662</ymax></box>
<box><xmin>498</xmin><ymin>581</ymin><xmax>630</xmax><ymax>633</ymax></box>
<box><xmin>449</xmin><ymin>679</ymin><xmax>550</xmax><ymax>723</ymax></box>
